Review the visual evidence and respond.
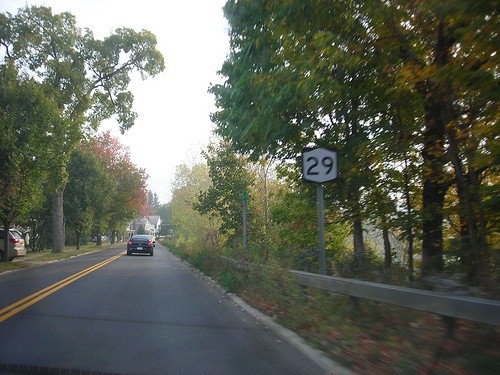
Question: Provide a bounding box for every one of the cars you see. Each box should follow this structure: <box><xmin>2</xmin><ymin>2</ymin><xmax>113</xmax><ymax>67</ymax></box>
<box><xmin>126</xmin><ymin>237</ymin><xmax>153</xmax><ymax>256</ymax></box>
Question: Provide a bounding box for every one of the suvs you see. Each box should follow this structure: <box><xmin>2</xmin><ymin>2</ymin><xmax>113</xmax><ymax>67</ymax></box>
<box><xmin>0</xmin><ymin>229</ymin><xmax>25</xmax><ymax>261</ymax></box>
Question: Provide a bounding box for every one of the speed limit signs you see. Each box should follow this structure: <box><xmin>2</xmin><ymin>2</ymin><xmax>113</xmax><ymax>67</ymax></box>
<box><xmin>301</xmin><ymin>147</ymin><xmax>338</xmax><ymax>184</ymax></box>
